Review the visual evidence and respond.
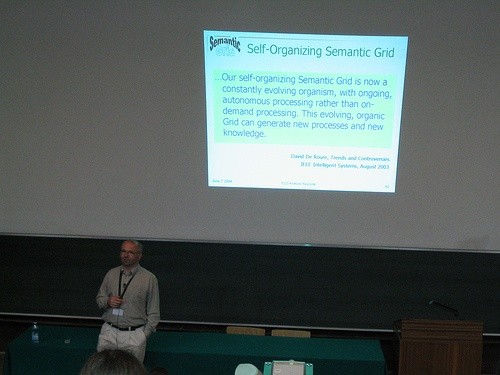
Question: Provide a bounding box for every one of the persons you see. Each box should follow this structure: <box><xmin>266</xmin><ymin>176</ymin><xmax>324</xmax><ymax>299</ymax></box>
<box><xmin>80</xmin><ymin>349</ymin><xmax>149</xmax><ymax>375</ymax></box>
<box><xmin>96</xmin><ymin>240</ymin><xmax>161</xmax><ymax>367</ymax></box>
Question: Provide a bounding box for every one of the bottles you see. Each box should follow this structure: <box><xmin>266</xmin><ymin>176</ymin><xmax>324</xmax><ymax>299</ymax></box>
<box><xmin>31</xmin><ymin>322</ymin><xmax>39</xmax><ymax>344</ymax></box>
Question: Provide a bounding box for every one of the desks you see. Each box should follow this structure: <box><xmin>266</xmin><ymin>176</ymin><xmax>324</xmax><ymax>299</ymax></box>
<box><xmin>3</xmin><ymin>324</ymin><xmax>388</xmax><ymax>375</ymax></box>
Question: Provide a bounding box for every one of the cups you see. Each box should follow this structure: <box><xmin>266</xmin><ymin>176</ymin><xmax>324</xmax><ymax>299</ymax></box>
<box><xmin>64</xmin><ymin>335</ymin><xmax>71</xmax><ymax>344</ymax></box>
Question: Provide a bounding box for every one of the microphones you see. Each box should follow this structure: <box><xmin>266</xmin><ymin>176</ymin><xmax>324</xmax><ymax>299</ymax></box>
<box><xmin>123</xmin><ymin>283</ymin><xmax>126</xmax><ymax>286</ymax></box>
<box><xmin>430</xmin><ymin>300</ymin><xmax>462</xmax><ymax>320</ymax></box>
